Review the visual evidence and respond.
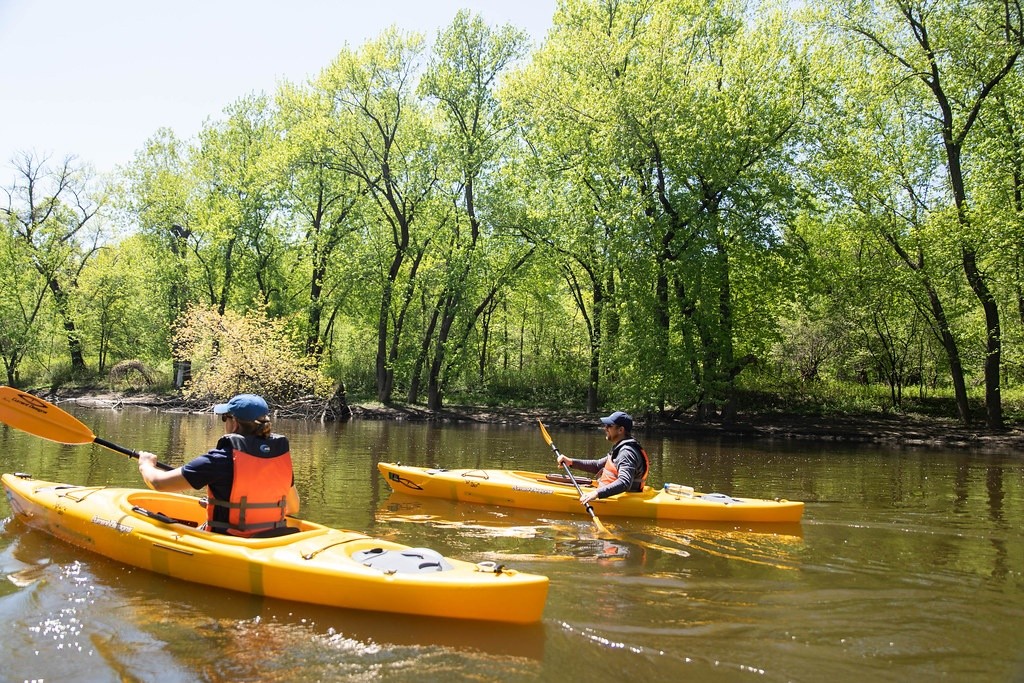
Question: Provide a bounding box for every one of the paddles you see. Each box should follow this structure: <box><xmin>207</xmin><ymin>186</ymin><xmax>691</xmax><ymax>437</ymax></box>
<box><xmin>1</xmin><ymin>385</ymin><xmax>175</xmax><ymax>471</ymax></box>
<box><xmin>538</xmin><ymin>417</ymin><xmax>614</xmax><ymax>535</ymax></box>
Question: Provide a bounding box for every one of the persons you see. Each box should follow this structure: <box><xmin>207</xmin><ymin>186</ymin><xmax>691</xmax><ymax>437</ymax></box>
<box><xmin>557</xmin><ymin>411</ymin><xmax>650</xmax><ymax>505</ymax></box>
<box><xmin>138</xmin><ymin>393</ymin><xmax>301</xmax><ymax>538</ymax></box>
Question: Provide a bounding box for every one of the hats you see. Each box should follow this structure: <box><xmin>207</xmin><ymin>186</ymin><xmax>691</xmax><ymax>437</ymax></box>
<box><xmin>600</xmin><ymin>412</ymin><xmax>632</xmax><ymax>432</ymax></box>
<box><xmin>214</xmin><ymin>394</ymin><xmax>269</xmax><ymax>424</ymax></box>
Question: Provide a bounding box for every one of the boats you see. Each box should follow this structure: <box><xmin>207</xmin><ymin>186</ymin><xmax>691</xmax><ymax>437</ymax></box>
<box><xmin>0</xmin><ymin>472</ymin><xmax>549</xmax><ymax>622</ymax></box>
<box><xmin>377</xmin><ymin>461</ymin><xmax>805</xmax><ymax>525</ymax></box>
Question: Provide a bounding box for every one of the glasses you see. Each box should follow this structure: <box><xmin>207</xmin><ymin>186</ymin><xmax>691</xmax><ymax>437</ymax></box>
<box><xmin>222</xmin><ymin>414</ymin><xmax>234</xmax><ymax>422</ymax></box>
<box><xmin>607</xmin><ymin>424</ymin><xmax>620</xmax><ymax>428</ymax></box>
